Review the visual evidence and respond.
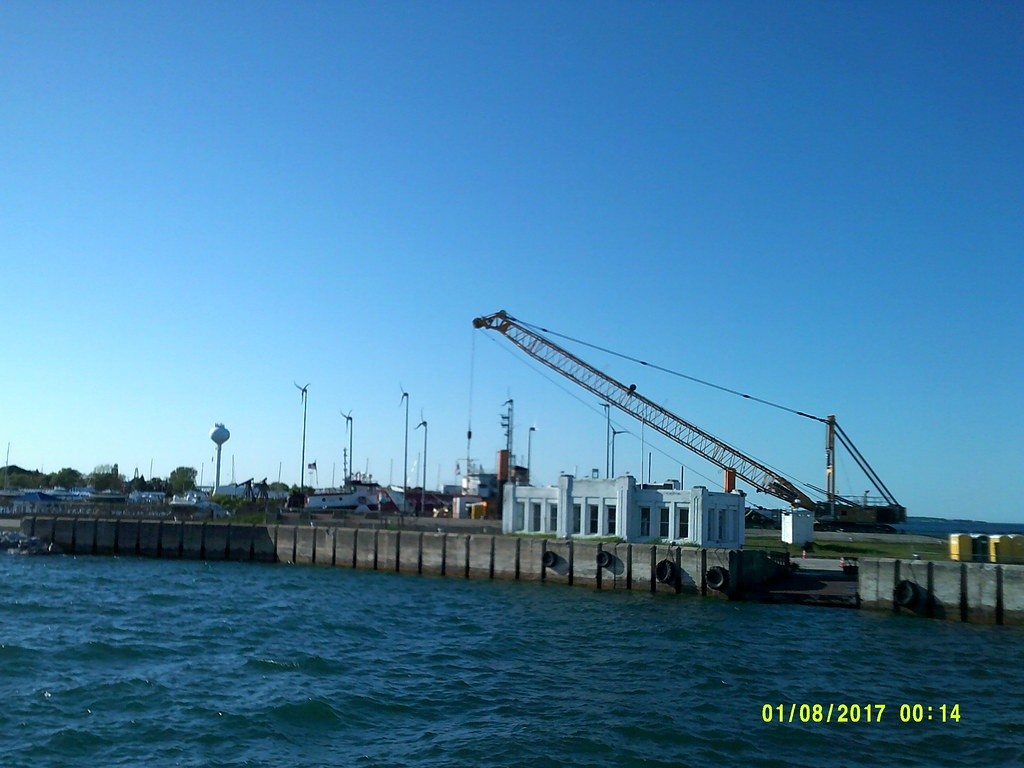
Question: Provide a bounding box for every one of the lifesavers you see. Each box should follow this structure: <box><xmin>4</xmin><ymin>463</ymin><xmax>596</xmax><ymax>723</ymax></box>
<box><xmin>541</xmin><ymin>551</ymin><xmax>557</xmax><ymax>567</ymax></box>
<box><xmin>654</xmin><ymin>559</ymin><xmax>677</xmax><ymax>584</ymax></box>
<box><xmin>705</xmin><ymin>567</ymin><xmax>731</xmax><ymax>589</ymax></box>
<box><xmin>595</xmin><ymin>550</ymin><xmax>613</xmax><ymax>568</ymax></box>
<box><xmin>891</xmin><ymin>580</ymin><xmax>917</xmax><ymax>606</ymax></box>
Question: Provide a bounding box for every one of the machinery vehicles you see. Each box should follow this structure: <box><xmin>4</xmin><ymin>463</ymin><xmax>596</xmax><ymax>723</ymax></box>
<box><xmin>471</xmin><ymin>310</ymin><xmax>907</xmax><ymax>534</ymax></box>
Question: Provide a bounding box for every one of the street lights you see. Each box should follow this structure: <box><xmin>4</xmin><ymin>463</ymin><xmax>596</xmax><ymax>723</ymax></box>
<box><xmin>527</xmin><ymin>427</ymin><xmax>535</xmax><ymax>482</ymax></box>
